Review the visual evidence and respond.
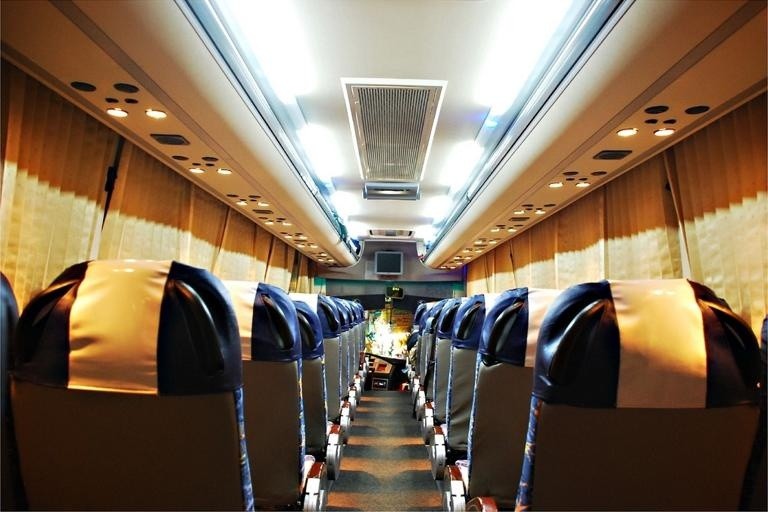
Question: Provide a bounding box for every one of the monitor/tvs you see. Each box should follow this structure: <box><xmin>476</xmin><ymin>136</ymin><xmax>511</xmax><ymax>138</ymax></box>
<box><xmin>374</xmin><ymin>251</ymin><xmax>404</xmax><ymax>276</ymax></box>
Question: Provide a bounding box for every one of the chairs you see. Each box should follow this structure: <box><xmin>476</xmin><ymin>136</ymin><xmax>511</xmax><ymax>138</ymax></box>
<box><xmin>759</xmin><ymin>314</ymin><xmax>767</xmax><ymax>512</ymax></box>
<box><xmin>0</xmin><ymin>273</ymin><xmax>19</xmax><ymax>512</ymax></box>
<box><xmin>223</xmin><ymin>279</ymin><xmax>370</xmax><ymax>512</ymax></box>
<box><xmin>403</xmin><ymin>287</ymin><xmax>558</xmax><ymax>512</ymax></box>
<box><xmin>10</xmin><ymin>257</ymin><xmax>256</xmax><ymax>512</ymax></box>
<box><xmin>465</xmin><ymin>277</ymin><xmax>757</xmax><ymax>512</ymax></box>
